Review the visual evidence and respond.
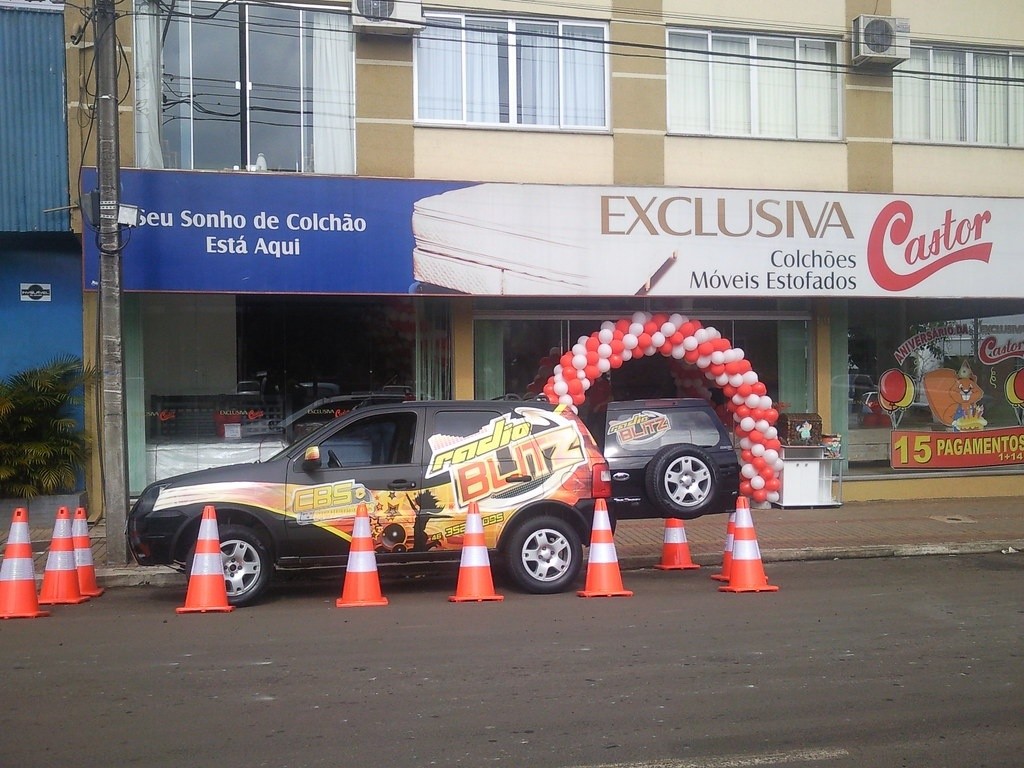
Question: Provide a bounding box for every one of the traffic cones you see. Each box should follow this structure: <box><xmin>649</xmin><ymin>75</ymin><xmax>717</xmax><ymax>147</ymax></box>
<box><xmin>0</xmin><ymin>507</ymin><xmax>50</xmax><ymax>620</ymax></box>
<box><xmin>39</xmin><ymin>506</ymin><xmax>89</xmax><ymax>605</ymax></box>
<box><xmin>70</xmin><ymin>507</ymin><xmax>105</xmax><ymax>597</ymax></box>
<box><xmin>337</xmin><ymin>504</ymin><xmax>389</xmax><ymax>609</ymax></box>
<box><xmin>176</xmin><ymin>505</ymin><xmax>237</xmax><ymax>613</ymax></box>
<box><xmin>711</xmin><ymin>511</ymin><xmax>769</xmax><ymax>580</ymax></box>
<box><xmin>575</xmin><ymin>498</ymin><xmax>633</xmax><ymax>598</ymax></box>
<box><xmin>719</xmin><ymin>496</ymin><xmax>778</xmax><ymax>594</ymax></box>
<box><xmin>655</xmin><ymin>518</ymin><xmax>701</xmax><ymax>570</ymax></box>
<box><xmin>448</xmin><ymin>502</ymin><xmax>504</xmax><ymax>601</ymax></box>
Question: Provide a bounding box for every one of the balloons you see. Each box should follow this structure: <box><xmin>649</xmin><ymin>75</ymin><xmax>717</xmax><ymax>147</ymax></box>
<box><xmin>539</xmin><ymin>312</ymin><xmax>784</xmax><ymax>510</ymax></box>
<box><xmin>525</xmin><ymin>338</ymin><xmax>570</xmax><ymax>396</ymax></box>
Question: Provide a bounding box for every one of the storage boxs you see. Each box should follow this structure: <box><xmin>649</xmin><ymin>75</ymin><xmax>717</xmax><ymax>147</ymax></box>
<box><xmin>778</xmin><ymin>410</ymin><xmax>825</xmax><ymax>447</ymax></box>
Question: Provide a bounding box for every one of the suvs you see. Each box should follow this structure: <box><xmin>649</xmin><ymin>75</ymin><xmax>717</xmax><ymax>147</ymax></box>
<box><xmin>525</xmin><ymin>397</ymin><xmax>741</xmax><ymax>526</ymax></box>
<box><xmin>125</xmin><ymin>397</ymin><xmax>620</xmax><ymax>608</ymax></box>
<box><xmin>226</xmin><ymin>386</ymin><xmax>415</xmax><ymax>444</ymax></box>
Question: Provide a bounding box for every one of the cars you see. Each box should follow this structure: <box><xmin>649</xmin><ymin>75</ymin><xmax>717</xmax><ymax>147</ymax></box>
<box><xmin>237</xmin><ymin>381</ymin><xmax>264</xmax><ymax>404</ymax></box>
<box><xmin>828</xmin><ymin>374</ymin><xmax>881</xmax><ymax>409</ymax></box>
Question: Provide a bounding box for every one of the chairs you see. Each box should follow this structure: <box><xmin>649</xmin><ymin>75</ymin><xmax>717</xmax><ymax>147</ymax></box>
<box><xmin>370</xmin><ymin>420</ymin><xmax>397</xmax><ymax>463</ymax></box>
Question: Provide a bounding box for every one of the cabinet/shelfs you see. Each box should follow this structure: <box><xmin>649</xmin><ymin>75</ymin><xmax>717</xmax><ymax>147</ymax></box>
<box><xmin>773</xmin><ymin>444</ymin><xmax>845</xmax><ymax>510</ymax></box>
